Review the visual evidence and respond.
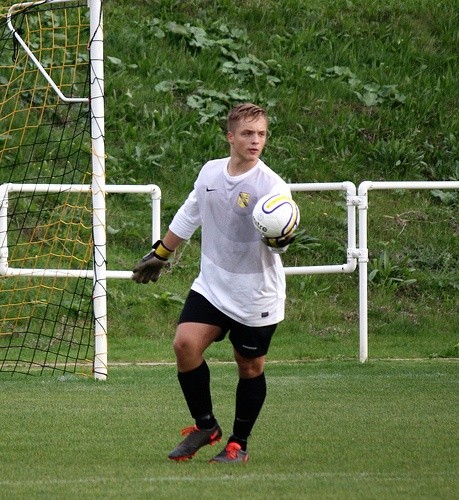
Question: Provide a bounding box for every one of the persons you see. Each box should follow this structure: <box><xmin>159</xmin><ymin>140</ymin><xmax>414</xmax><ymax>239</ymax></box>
<box><xmin>132</xmin><ymin>101</ymin><xmax>297</xmax><ymax>469</ymax></box>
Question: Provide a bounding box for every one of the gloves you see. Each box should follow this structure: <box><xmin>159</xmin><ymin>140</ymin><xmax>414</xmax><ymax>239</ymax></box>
<box><xmin>261</xmin><ymin>235</ymin><xmax>297</xmax><ymax>254</ymax></box>
<box><xmin>131</xmin><ymin>240</ymin><xmax>175</xmax><ymax>284</ymax></box>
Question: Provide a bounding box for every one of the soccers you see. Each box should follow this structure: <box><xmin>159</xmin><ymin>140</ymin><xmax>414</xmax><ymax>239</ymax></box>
<box><xmin>251</xmin><ymin>192</ymin><xmax>301</xmax><ymax>238</ymax></box>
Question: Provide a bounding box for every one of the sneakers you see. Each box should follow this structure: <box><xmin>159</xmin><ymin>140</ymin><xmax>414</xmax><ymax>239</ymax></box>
<box><xmin>168</xmin><ymin>421</ymin><xmax>222</xmax><ymax>461</ymax></box>
<box><xmin>209</xmin><ymin>441</ymin><xmax>249</xmax><ymax>464</ymax></box>
